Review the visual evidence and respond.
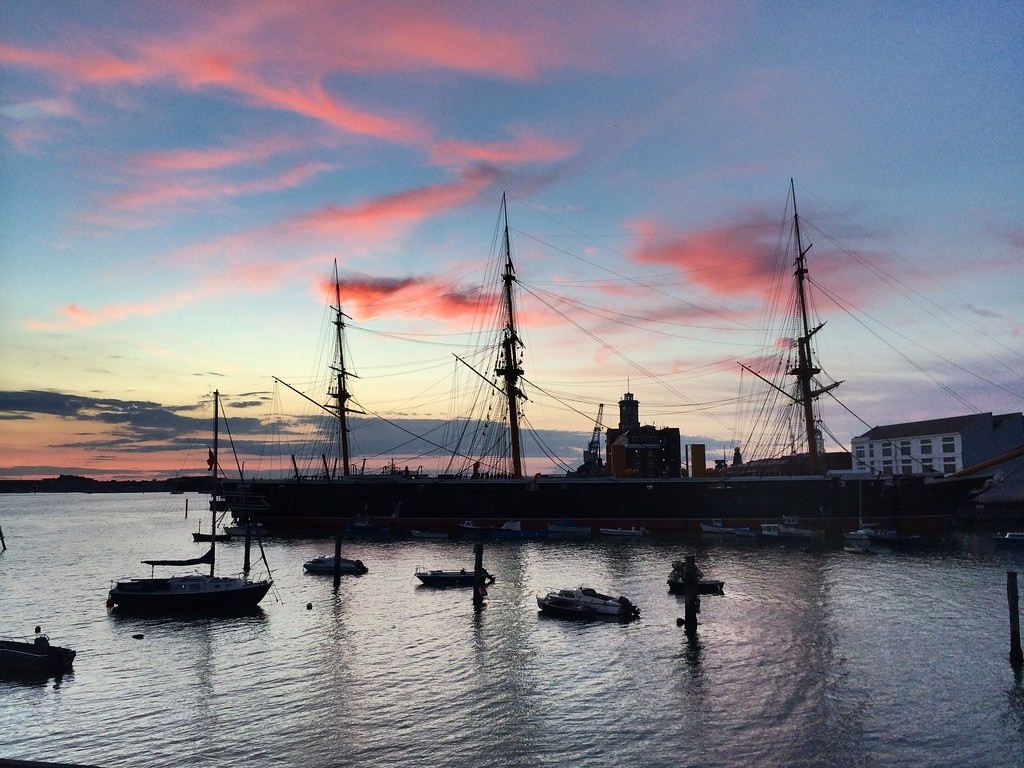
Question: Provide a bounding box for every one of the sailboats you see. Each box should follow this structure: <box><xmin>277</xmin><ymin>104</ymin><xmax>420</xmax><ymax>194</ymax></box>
<box><xmin>216</xmin><ymin>174</ymin><xmax>1024</xmax><ymax>529</ymax></box>
<box><xmin>106</xmin><ymin>388</ymin><xmax>274</xmax><ymax>609</ymax></box>
<box><xmin>859</xmin><ymin>478</ymin><xmax>881</xmax><ymax>530</ymax></box>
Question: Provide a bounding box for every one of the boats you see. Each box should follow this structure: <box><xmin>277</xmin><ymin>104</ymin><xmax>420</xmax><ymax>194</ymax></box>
<box><xmin>192</xmin><ymin>533</ymin><xmax>233</xmax><ymax>542</ymax></box>
<box><xmin>545</xmin><ymin>517</ymin><xmax>592</xmax><ymax>533</ymax></box>
<box><xmin>668</xmin><ymin>578</ymin><xmax>725</xmax><ymax>593</ymax></box>
<box><xmin>0</xmin><ymin>640</ymin><xmax>77</xmax><ymax>673</ymax></box>
<box><xmin>844</xmin><ymin>547</ymin><xmax>864</xmax><ymax>553</ymax></box>
<box><xmin>759</xmin><ymin>513</ymin><xmax>826</xmax><ymax>538</ymax></box>
<box><xmin>535</xmin><ymin>582</ymin><xmax>641</xmax><ymax>619</ymax></box>
<box><xmin>224</xmin><ymin>525</ymin><xmax>268</xmax><ymax>536</ymax></box>
<box><xmin>599</xmin><ymin>525</ymin><xmax>650</xmax><ymax>536</ymax></box>
<box><xmin>698</xmin><ymin>523</ymin><xmax>735</xmax><ymax>534</ymax></box>
<box><xmin>994</xmin><ymin>531</ymin><xmax>1024</xmax><ymax>543</ymax></box>
<box><xmin>410</xmin><ymin>530</ymin><xmax>450</xmax><ymax>538</ymax></box>
<box><xmin>414</xmin><ymin>566</ymin><xmax>496</xmax><ymax>584</ymax></box>
<box><xmin>456</xmin><ymin>520</ymin><xmax>549</xmax><ymax>540</ymax></box>
<box><xmin>302</xmin><ymin>555</ymin><xmax>369</xmax><ymax>574</ymax></box>
<box><xmin>865</xmin><ymin>532</ymin><xmax>921</xmax><ymax>544</ymax></box>
<box><xmin>734</xmin><ymin>529</ymin><xmax>758</xmax><ymax>536</ymax></box>
